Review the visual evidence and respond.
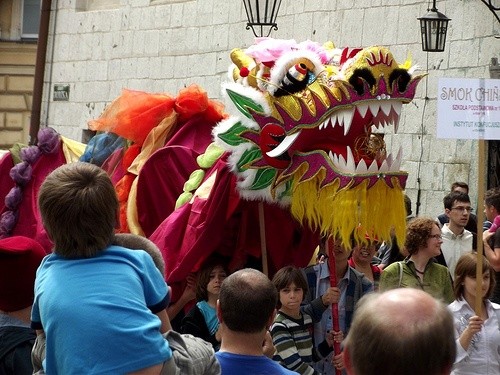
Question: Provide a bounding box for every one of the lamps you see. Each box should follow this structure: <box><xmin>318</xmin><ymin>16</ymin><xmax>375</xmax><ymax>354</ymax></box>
<box><xmin>242</xmin><ymin>0</ymin><xmax>281</xmax><ymax>38</ymax></box>
<box><xmin>417</xmin><ymin>0</ymin><xmax>452</xmax><ymax>52</ymax></box>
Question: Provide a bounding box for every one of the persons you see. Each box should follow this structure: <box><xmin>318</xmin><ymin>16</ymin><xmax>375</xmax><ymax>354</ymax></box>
<box><xmin>0</xmin><ymin>182</ymin><xmax>500</xmax><ymax>375</ymax></box>
<box><xmin>30</xmin><ymin>162</ymin><xmax>222</xmax><ymax>375</ymax></box>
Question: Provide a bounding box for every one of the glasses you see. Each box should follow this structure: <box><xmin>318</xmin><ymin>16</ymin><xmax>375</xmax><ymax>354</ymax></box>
<box><xmin>429</xmin><ymin>233</ymin><xmax>443</xmax><ymax>240</ymax></box>
<box><xmin>452</xmin><ymin>206</ymin><xmax>475</xmax><ymax>213</ymax></box>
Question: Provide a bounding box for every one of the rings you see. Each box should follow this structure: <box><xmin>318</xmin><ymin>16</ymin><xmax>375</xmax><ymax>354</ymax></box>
<box><xmin>337</xmin><ymin>363</ymin><xmax>341</xmax><ymax>367</ymax></box>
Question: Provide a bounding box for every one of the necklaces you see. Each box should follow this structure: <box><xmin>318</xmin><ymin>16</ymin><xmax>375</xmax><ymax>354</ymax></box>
<box><xmin>416</xmin><ymin>268</ymin><xmax>424</xmax><ymax>274</ymax></box>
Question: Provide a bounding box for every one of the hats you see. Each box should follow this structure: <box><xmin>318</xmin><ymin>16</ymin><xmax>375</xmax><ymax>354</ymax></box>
<box><xmin>351</xmin><ymin>225</ymin><xmax>384</xmax><ymax>243</ymax></box>
